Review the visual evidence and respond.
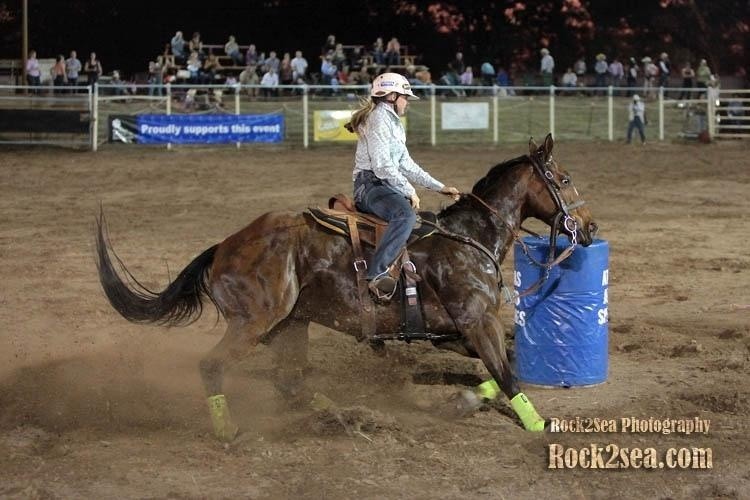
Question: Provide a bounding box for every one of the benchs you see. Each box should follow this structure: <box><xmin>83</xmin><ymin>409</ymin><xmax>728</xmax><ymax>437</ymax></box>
<box><xmin>162</xmin><ymin>43</ymin><xmax>422</xmax><ymax>71</ymax></box>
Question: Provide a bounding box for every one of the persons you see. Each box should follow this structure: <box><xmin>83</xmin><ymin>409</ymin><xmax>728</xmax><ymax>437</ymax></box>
<box><xmin>344</xmin><ymin>73</ymin><xmax>460</xmax><ymax>304</ymax></box>
<box><xmin>24</xmin><ymin>48</ymin><xmax>127</xmax><ymax>96</ymax></box>
<box><xmin>627</xmin><ymin>94</ymin><xmax>648</xmax><ymax>145</ymax></box>
<box><xmin>150</xmin><ymin>35</ymin><xmax>749</xmax><ymax>96</ymax></box>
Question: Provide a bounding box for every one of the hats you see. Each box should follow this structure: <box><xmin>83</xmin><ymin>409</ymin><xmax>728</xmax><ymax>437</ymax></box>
<box><xmin>596</xmin><ymin>52</ymin><xmax>668</xmax><ymax>63</ymax></box>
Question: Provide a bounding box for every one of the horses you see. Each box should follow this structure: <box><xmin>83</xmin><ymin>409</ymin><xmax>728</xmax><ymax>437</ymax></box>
<box><xmin>90</xmin><ymin>132</ymin><xmax>598</xmax><ymax>444</ymax></box>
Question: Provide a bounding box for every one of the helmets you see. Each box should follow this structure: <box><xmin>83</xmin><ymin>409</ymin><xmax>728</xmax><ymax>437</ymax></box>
<box><xmin>632</xmin><ymin>94</ymin><xmax>640</xmax><ymax>101</ymax></box>
<box><xmin>371</xmin><ymin>72</ymin><xmax>420</xmax><ymax>102</ymax></box>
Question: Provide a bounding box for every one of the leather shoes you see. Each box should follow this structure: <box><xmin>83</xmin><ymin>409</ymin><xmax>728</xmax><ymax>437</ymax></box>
<box><xmin>368</xmin><ymin>276</ymin><xmax>396</xmax><ymax>297</ymax></box>
